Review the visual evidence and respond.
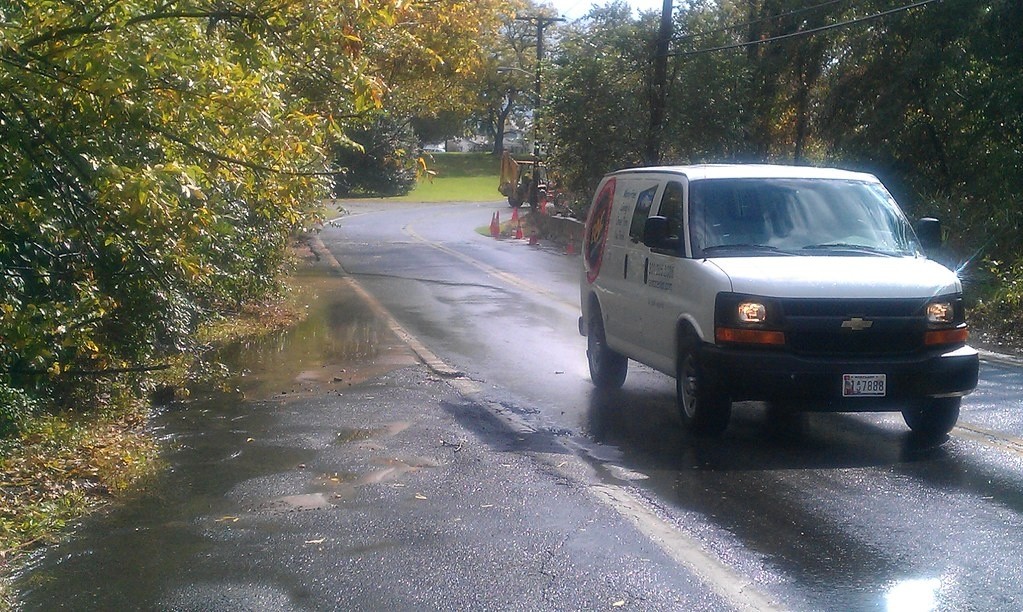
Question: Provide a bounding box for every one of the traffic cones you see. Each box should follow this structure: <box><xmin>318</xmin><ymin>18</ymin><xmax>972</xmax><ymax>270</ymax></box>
<box><xmin>562</xmin><ymin>229</ymin><xmax>578</xmax><ymax>255</ymax></box>
<box><xmin>512</xmin><ymin>220</ymin><xmax>525</xmax><ymax>240</ymax></box>
<box><xmin>510</xmin><ymin>207</ymin><xmax>520</xmax><ymax>221</ymax></box>
<box><xmin>527</xmin><ymin>223</ymin><xmax>539</xmax><ymax>246</ymax></box>
<box><xmin>490</xmin><ymin>211</ymin><xmax>502</xmax><ymax>238</ymax></box>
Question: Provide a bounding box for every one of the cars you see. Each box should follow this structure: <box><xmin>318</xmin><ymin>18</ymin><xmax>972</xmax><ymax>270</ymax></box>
<box><xmin>467</xmin><ymin>144</ymin><xmax>491</xmax><ymax>152</ymax></box>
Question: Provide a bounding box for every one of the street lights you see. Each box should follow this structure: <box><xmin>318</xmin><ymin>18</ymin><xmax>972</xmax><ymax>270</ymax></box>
<box><xmin>496</xmin><ymin>67</ymin><xmax>541</xmax><ymax>210</ymax></box>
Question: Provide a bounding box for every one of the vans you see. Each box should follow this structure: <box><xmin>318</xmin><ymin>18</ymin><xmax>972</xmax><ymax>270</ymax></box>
<box><xmin>578</xmin><ymin>164</ymin><xmax>979</xmax><ymax>439</ymax></box>
